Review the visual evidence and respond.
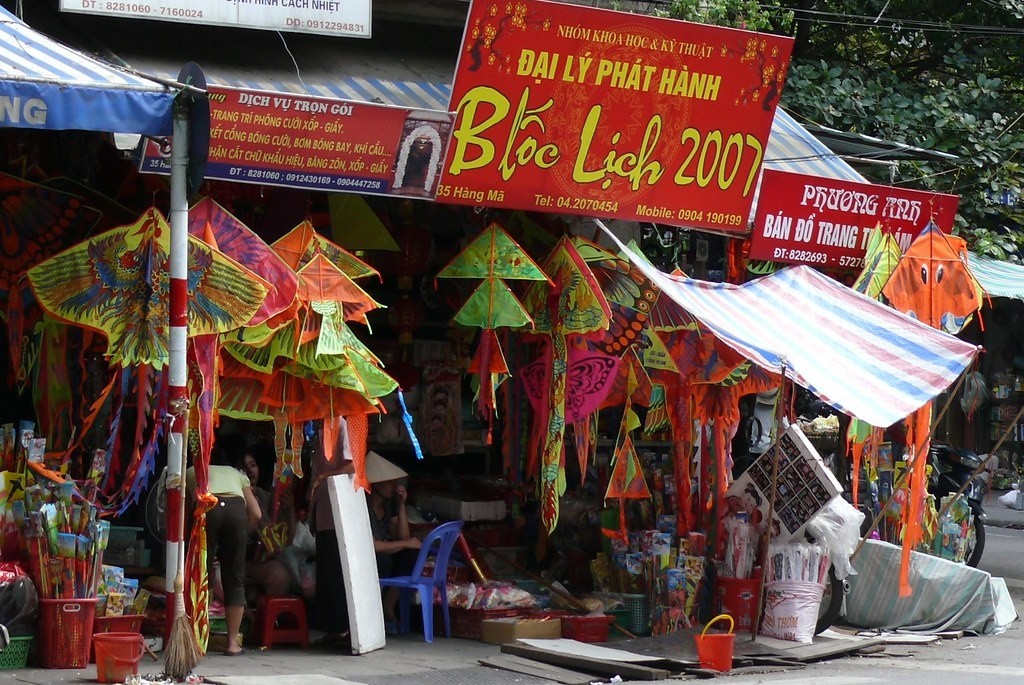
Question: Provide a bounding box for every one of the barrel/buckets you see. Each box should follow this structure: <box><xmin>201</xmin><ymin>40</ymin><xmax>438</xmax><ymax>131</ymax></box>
<box><xmin>694</xmin><ymin>614</ymin><xmax>735</xmax><ymax>672</ymax></box>
<box><xmin>711</xmin><ymin>575</ymin><xmax>765</xmax><ymax>633</ymax></box>
<box><xmin>92</xmin><ymin>633</ymin><xmax>144</xmax><ymax>683</ymax></box>
<box><xmin>95</xmin><ymin>615</ymin><xmax>144</xmax><ymax>633</ymax></box>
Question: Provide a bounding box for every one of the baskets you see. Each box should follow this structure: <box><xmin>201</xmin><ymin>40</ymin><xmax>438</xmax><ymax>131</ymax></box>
<box><xmin>39</xmin><ymin>598</ymin><xmax>101</xmax><ymax>669</ymax></box>
<box><xmin>590</xmin><ymin>591</ymin><xmax>649</xmax><ymax>634</ymax></box>
<box><xmin>0</xmin><ymin>636</ymin><xmax>33</xmax><ymax>669</ymax></box>
<box><xmin>209</xmin><ymin>616</ymin><xmax>228</xmax><ymax>632</ymax></box>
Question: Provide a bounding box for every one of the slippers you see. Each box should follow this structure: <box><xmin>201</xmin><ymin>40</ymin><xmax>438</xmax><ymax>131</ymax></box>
<box><xmin>224</xmin><ymin>644</ymin><xmax>245</xmax><ymax>655</ymax></box>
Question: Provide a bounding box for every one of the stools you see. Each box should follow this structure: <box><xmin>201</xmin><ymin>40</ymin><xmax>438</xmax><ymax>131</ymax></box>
<box><xmin>250</xmin><ymin>595</ymin><xmax>308</xmax><ymax>652</ymax></box>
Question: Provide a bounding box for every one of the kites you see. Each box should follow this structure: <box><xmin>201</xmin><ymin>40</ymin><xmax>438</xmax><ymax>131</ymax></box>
<box><xmin>0</xmin><ymin>174</ymin><xmax>993</xmax><ymax>656</ymax></box>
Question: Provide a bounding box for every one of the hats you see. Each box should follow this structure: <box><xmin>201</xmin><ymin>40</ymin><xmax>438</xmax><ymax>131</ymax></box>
<box><xmin>365</xmin><ymin>451</ymin><xmax>408</xmax><ymax>483</ymax></box>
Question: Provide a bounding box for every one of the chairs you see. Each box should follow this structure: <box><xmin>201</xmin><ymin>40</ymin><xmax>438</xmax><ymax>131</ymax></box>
<box><xmin>379</xmin><ymin>520</ymin><xmax>464</xmax><ymax>642</ymax></box>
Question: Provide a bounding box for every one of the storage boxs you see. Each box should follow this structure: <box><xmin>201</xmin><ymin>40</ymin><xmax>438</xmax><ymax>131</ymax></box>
<box><xmin>102</xmin><ymin>524</ymin><xmax>144</xmax><ymax>566</ymax></box>
<box><xmin>481</xmin><ymin>616</ymin><xmax>561</xmax><ymax>646</ymax></box>
<box><xmin>90</xmin><ymin>613</ymin><xmax>146</xmax><ymax>663</ymax></box>
<box><xmin>561</xmin><ymin>615</ymin><xmax>615</xmax><ymax>642</ymax></box>
<box><xmin>413</xmin><ymin>491</ymin><xmax>504</xmax><ymax>519</ymax></box>
<box><xmin>567</xmin><ymin>608</ymin><xmax>631</xmax><ymax>638</ymax></box>
<box><xmin>478</xmin><ymin>546</ymin><xmax>528</xmax><ymax>576</ymax></box>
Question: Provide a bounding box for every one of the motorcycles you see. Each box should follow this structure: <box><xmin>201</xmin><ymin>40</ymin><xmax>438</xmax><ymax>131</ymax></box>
<box><xmin>928</xmin><ymin>443</ymin><xmax>987</xmax><ymax>569</ymax></box>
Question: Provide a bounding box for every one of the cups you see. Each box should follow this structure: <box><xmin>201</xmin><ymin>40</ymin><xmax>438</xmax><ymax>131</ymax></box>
<box><xmin>137</xmin><ymin>549</ymin><xmax>151</xmax><ymax>568</ymax></box>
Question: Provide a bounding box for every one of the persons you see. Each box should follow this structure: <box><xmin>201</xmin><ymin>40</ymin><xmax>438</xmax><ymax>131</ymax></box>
<box><xmin>236</xmin><ymin>450</ymin><xmax>291</xmax><ymax>639</ymax></box>
<box><xmin>186</xmin><ymin>458</ymin><xmax>261</xmax><ymax>657</ymax></box>
<box><xmin>363</xmin><ymin>452</ymin><xmax>422</xmax><ymax>624</ymax></box>
